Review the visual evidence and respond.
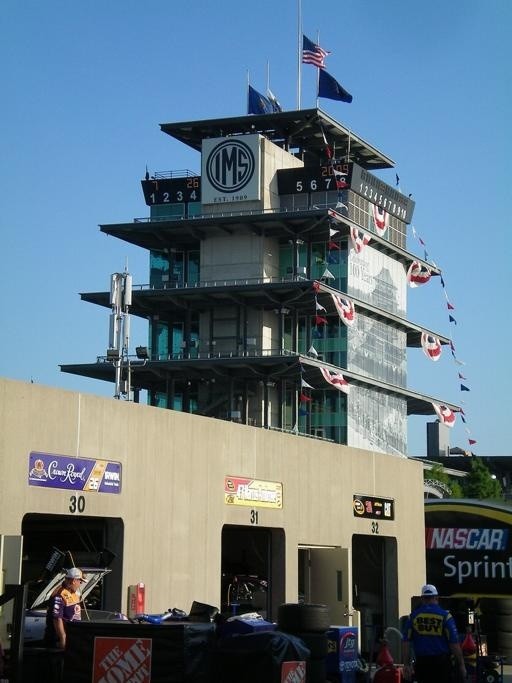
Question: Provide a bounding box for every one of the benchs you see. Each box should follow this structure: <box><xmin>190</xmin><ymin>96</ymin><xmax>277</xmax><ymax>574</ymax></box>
<box><xmin>300</xmin><ymin>33</ymin><xmax>332</xmax><ymax>68</ymax></box>
<box><xmin>317</xmin><ymin>66</ymin><xmax>353</xmax><ymax>104</ymax></box>
<box><xmin>265</xmin><ymin>88</ymin><xmax>281</xmax><ymax>111</ymax></box>
<box><xmin>248</xmin><ymin>83</ymin><xmax>273</xmax><ymax>114</ymax></box>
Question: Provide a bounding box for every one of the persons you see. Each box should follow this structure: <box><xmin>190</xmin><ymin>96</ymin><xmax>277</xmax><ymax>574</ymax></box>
<box><xmin>401</xmin><ymin>583</ymin><xmax>469</xmax><ymax>682</ymax></box>
<box><xmin>43</xmin><ymin>566</ymin><xmax>87</xmax><ymax>647</ymax></box>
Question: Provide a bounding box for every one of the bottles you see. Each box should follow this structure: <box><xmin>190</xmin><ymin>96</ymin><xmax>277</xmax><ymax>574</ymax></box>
<box><xmin>421</xmin><ymin>584</ymin><xmax>438</xmax><ymax>595</ymax></box>
<box><xmin>65</xmin><ymin>567</ymin><xmax>88</xmax><ymax>583</ymax></box>
